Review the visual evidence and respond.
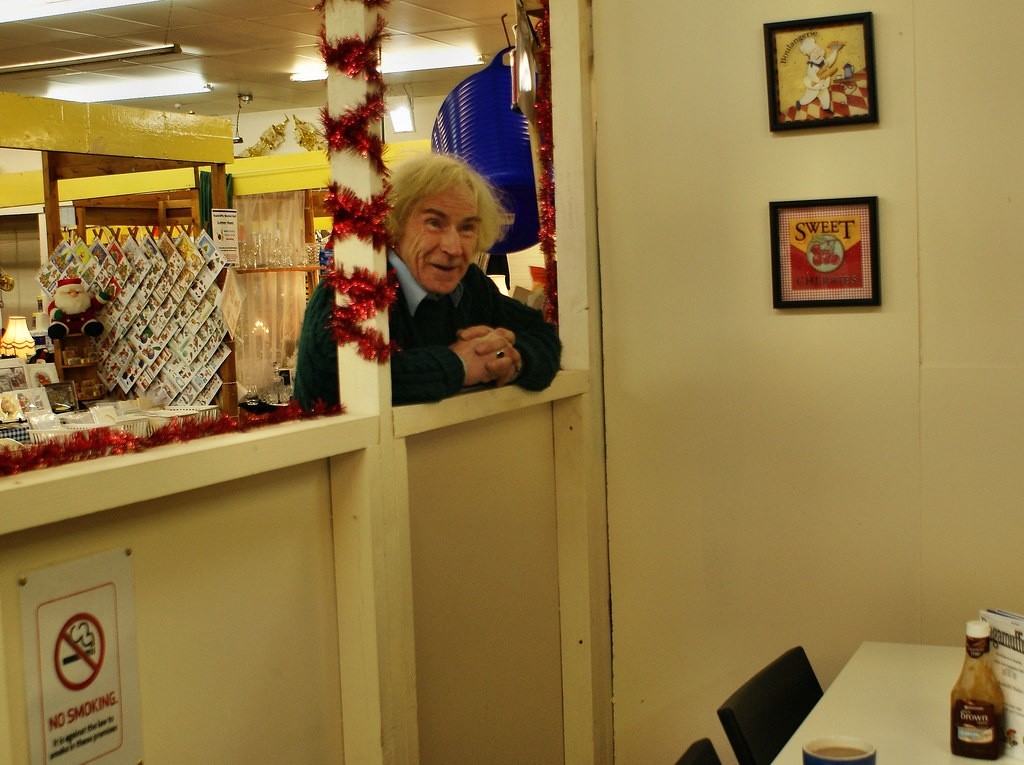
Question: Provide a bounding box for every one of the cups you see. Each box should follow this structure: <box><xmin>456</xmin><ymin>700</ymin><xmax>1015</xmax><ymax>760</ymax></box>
<box><xmin>244</xmin><ymin>384</ymin><xmax>259</xmax><ymax>406</ymax></box>
<box><xmin>260</xmin><ymin>387</ymin><xmax>294</xmax><ymax>404</ymax></box>
<box><xmin>240</xmin><ymin>234</ymin><xmax>320</xmax><ymax>270</ymax></box>
<box><xmin>803</xmin><ymin>735</ymin><xmax>876</xmax><ymax>764</ymax></box>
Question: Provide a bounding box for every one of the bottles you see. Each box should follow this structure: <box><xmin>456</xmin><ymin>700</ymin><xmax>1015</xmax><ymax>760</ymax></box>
<box><xmin>269</xmin><ymin>362</ymin><xmax>284</xmax><ymax>392</ymax></box>
<box><xmin>950</xmin><ymin>620</ymin><xmax>1009</xmax><ymax>759</ymax></box>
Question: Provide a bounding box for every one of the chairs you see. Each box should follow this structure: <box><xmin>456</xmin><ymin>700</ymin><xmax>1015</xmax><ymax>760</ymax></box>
<box><xmin>718</xmin><ymin>645</ymin><xmax>825</xmax><ymax>765</ymax></box>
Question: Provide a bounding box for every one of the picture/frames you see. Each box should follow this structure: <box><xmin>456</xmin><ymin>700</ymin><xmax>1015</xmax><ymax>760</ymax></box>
<box><xmin>767</xmin><ymin>195</ymin><xmax>884</xmax><ymax>310</ymax></box>
<box><xmin>761</xmin><ymin>11</ymin><xmax>878</xmax><ymax>133</ymax></box>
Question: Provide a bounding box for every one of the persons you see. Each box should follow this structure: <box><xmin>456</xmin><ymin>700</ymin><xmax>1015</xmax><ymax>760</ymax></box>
<box><xmin>292</xmin><ymin>152</ymin><xmax>564</xmax><ymax>412</ymax></box>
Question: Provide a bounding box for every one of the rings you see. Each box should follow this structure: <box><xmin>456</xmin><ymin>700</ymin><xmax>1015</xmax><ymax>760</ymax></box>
<box><xmin>496</xmin><ymin>350</ymin><xmax>505</xmax><ymax>358</ymax></box>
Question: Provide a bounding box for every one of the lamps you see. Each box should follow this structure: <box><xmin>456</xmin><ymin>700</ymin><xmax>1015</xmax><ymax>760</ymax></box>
<box><xmin>1</xmin><ymin>1</ymin><xmax>183</xmax><ymax>79</ymax></box>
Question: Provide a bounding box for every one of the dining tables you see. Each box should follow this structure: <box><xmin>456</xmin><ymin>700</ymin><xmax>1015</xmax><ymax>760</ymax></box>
<box><xmin>770</xmin><ymin>639</ymin><xmax>1024</xmax><ymax>765</ymax></box>
